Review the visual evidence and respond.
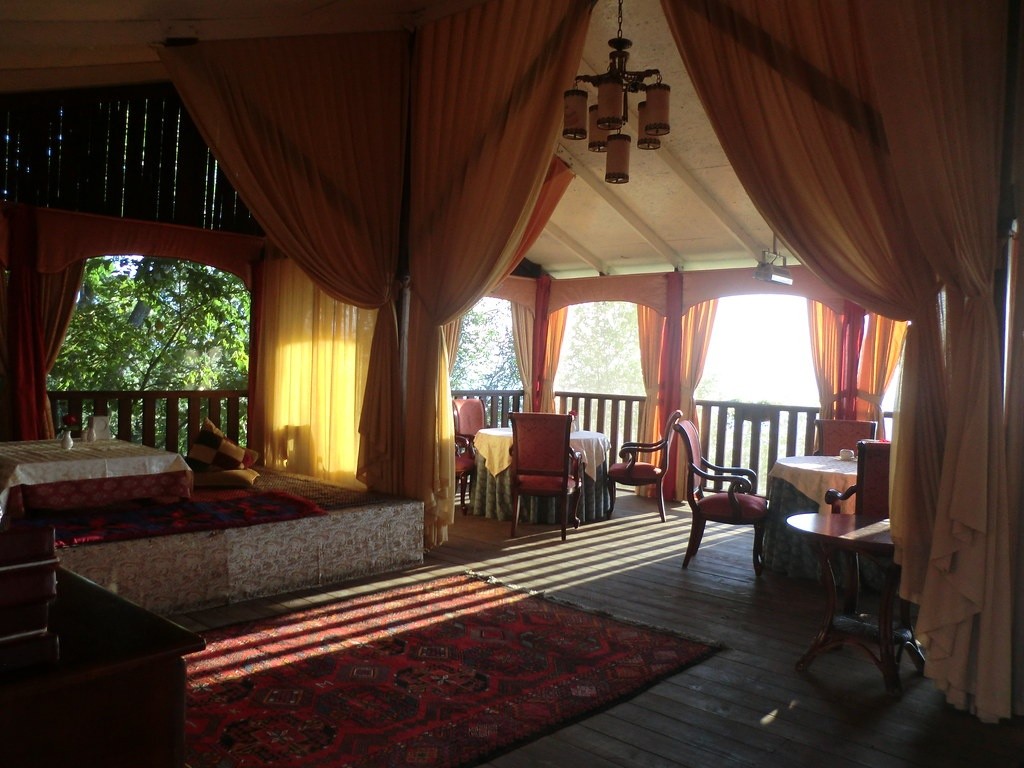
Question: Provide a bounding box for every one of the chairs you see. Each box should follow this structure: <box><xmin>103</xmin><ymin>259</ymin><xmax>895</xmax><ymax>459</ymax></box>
<box><xmin>825</xmin><ymin>443</ymin><xmax>890</xmax><ymax>514</ymax></box>
<box><xmin>674</xmin><ymin>419</ymin><xmax>770</xmax><ymax>576</ymax></box>
<box><xmin>509</xmin><ymin>412</ymin><xmax>582</xmax><ymax>541</ymax></box>
<box><xmin>814</xmin><ymin>420</ymin><xmax>877</xmax><ymax>455</ymax></box>
<box><xmin>453</xmin><ymin>398</ymin><xmax>491</xmax><ymax>516</ymax></box>
<box><xmin>607</xmin><ymin>410</ymin><xmax>683</xmax><ymax>522</ymax></box>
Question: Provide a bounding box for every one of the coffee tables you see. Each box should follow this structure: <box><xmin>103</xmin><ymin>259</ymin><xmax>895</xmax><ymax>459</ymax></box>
<box><xmin>0</xmin><ymin>437</ymin><xmax>192</xmax><ymax>534</ymax></box>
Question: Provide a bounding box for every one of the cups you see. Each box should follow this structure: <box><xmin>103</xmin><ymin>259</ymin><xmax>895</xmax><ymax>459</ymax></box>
<box><xmin>81</xmin><ymin>431</ymin><xmax>87</xmax><ymax>442</ymax></box>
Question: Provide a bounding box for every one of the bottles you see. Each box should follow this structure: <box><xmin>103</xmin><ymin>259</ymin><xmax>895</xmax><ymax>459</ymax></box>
<box><xmin>856</xmin><ymin>441</ymin><xmax>865</xmax><ymax>459</ymax></box>
<box><xmin>87</xmin><ymin>424</ymin><xmax>96</xmax><ymax>442</ymax></box>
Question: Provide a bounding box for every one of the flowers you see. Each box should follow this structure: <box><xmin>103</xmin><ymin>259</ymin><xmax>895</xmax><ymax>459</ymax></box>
<box><xmin>61</xmin><ymin>414</ymin><xmax>82</xmax><ymax>431</ymax></box>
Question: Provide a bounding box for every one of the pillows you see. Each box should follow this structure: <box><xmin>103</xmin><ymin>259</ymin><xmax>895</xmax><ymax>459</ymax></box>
<box><xmin>185</xmin><ymin>417</ymin><xmax>258</xmax><ymax>472</ymax></box>
<box><xmin>194</xmin><ymin>469</ymin><xmax>260</xmax><ymax>487</ymax></box>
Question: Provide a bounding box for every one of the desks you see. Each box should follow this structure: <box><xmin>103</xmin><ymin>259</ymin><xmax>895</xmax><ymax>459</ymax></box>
<box><xmin>763</xmin><ymin>455</ymin><xmax>896</xmax><ymax>592</ymax></box>
<box><xmin>471</xmin><ymin>427</ymin><xmax>611</xmax><ymax>524</ymax></box>
<box><xmin>785</xmin><ymin>513</ymin><xmax>925</xmax><ymax>696</ymax></box>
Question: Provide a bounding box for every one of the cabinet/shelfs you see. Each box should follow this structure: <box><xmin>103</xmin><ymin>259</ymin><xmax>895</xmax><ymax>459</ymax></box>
<box><xmin>0</xmin><ymin>563</ymin><xmax>207</xmax><ymax>768</ymax></box>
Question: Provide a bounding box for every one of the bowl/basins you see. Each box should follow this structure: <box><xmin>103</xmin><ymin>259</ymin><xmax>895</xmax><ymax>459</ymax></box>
<box><xmin>840</xmin><ymin>450</ymin><xmax>853</xmax><ymax>460</ymax></box>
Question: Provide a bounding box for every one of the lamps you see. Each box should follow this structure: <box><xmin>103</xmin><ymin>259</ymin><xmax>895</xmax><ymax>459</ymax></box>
<box><xmin>563</xmin><ymin>0</ymin><xmax>670</xmax><ymax>184</ymax></box>
<box><xmin>752</xmin><ymin>232</ymin><xmax>793</xmax><ymax>286</ymax></box>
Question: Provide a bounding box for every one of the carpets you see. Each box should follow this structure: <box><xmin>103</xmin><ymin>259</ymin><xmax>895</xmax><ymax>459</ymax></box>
<box><xmin>179</xmin><ymin>570</ymin><xmax>730</xmax><ymax>768</ymax></box>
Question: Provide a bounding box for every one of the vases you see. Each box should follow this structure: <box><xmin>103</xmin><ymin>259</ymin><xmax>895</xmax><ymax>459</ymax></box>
<box><xmin>61</xmin><ymin>431</ymin><xmax>74</xmax><ymax>449</ymax></box>
